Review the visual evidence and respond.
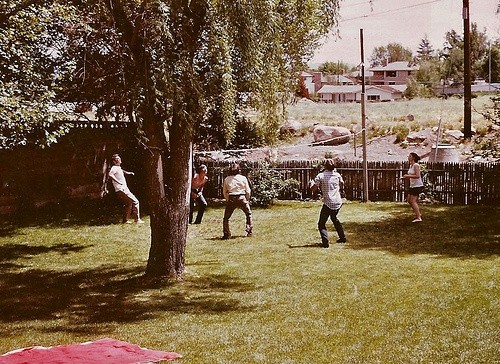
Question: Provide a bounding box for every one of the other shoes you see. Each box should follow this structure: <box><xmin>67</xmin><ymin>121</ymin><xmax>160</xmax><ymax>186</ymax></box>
<box><xmin>318</xmin><ymin>242</ymin><xmax>330</xmax><ymax>248</ymax></box>
<box><xmin>246</xmin><ymin>229</ymin><xmax>252</xmax><ymax>236</ymax></box>
<box><xmin>121</xmin><ymin>221</ymin><xmax>132</xmax><ymax>224</ymax></box>
<box><xmin>335</xmin><ymin>238</ymin><xmax>347</xmax><ymax>243</ymax></box>
<box><xmin>222</xmin><ymin>233</ymin><xmax>230</xmax><ymax>240</ymax></box>
<box><xmin>133</xmin><ymin>220</ymin><xmax>145</xmax><ymax>224</ymax></box>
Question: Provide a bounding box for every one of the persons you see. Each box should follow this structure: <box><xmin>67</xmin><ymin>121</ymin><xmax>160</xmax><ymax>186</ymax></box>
<box><xmin>402</xmin><ymin>153</ymin><xmax>424</xmax><ymax>223</ymax></box>
<box><xmin>311</xmin><ymin>159</ymin><xmax>347</xmax><ymax>247</ymax></box>
<box><xmin>108</xmin><ymin>154</ymin><xmax>144</xmax><ymax>223</ymax></box>
<box><xmin>221</xmin><ymin>163</ymin><xmax>254</xmax><ymax>239</ymax></box>
<box><xmin>190</xmin><ymin>164</ymin><xmax>209</xmax><ymax>225</ymax></box>
